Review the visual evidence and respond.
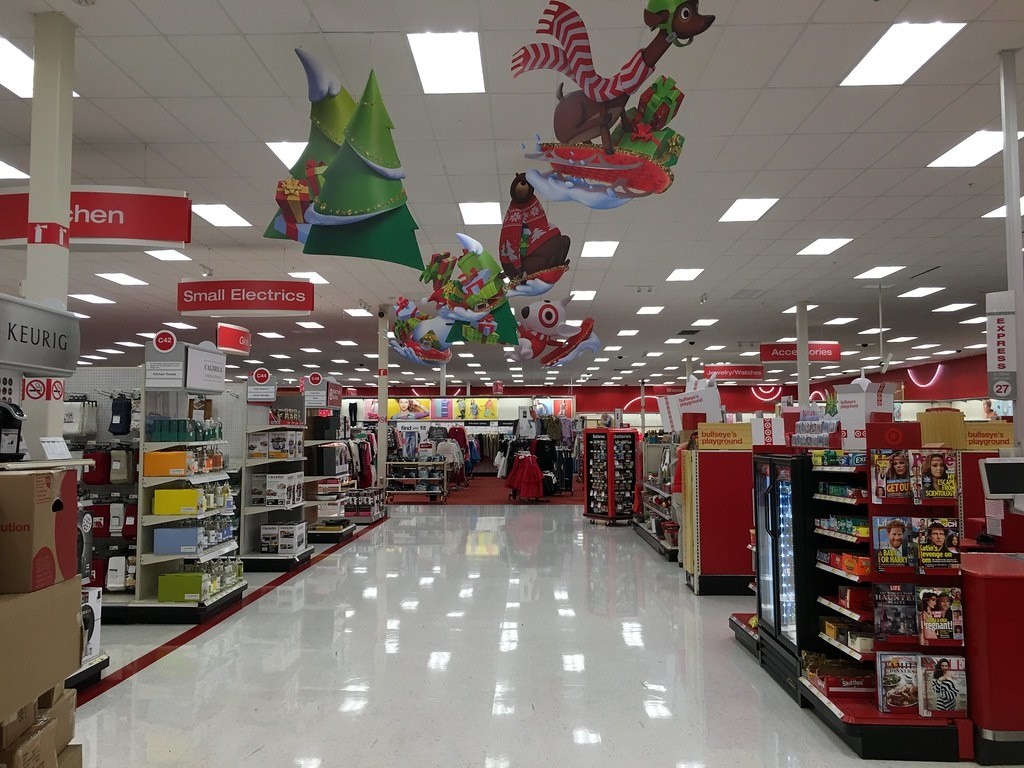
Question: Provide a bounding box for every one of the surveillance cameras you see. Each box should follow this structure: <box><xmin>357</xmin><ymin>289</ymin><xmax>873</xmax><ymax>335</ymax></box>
<box><xmin>359</xmin><ymin>364</ymin><xmax>364</xmax><ymax>366</ymax></box>
<box><xmin>956</xmin><ymin>350</ymin><xmax>961</xmax><ymax>353</ymax></box>
<box><xmin>547</xmin><ymin>385</ymin><xmax>549</xmax><ymax>386</ymax></box>
<box><xmin>812</xmin><ymin>378</ymin><xmax>815</xmax><ymax>380</ymax></box>
<box><xmin>783</xmin><ymin>384</ymin><xmax>785</xmax><ymax>385</ymax></box>
<box><xmin>378</xmin><ymin>311</ymin><xmax>385</xmax><ymax>318</ymax></box>
<box><xmin>618</xmin><ymin>356</ymin><xmax>623</xmax><ymax>359</ymax></box>
<box><xmin>843</xmin><ymin>372</ymin><xmax>846</xmax><ymax>374</ymax></box>
<box><xmin>862</xmin><ymin>344</ymin><xmax>868</xmax><ymax>347</ymax></box>
<box><xmin>689</xmin><ymin>342</ymin><xmax>695</xmax><ymax>345</ymax></box>
<box><xmin>625</xmin><ymin>385</ymin><xmax>628</xmax><ymax>386</ymax></box>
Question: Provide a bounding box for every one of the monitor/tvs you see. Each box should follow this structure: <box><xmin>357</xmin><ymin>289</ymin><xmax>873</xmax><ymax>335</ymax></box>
<box><xmin>881</xmin><ymin>353</ymin><xmax>893</xmax><ymax>374</ymax></box>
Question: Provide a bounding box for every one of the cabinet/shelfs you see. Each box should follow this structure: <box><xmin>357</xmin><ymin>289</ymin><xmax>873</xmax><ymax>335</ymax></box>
<box><xmin>386</xmin><ymin>461</ymin><xmax>448</xmax><ymax>503</ymax></box>
<box><xmin>244</xmin><ymin>423</ymin><xmax>315</xmax><ymax>569</ymax></box>
<box><xmin>797</xmin><ymin>422</ymin><xmax>924</xmax><ymax>737</ymax></box>
<box><xmin>642</xmin><ymin>482</ymin><xmax>679</xmax><ymax>563</ymax></box>
<box><xmin>137</xmin><ymin>365</ymin><xmax>248</xmax><ymax>619</ymax></box>
<box><xmin>304</xmin><ymin>437</ymin><xmax>355</xmax><ymax>541</ymax></box>
<box><xmin>730</xmin><ymin>542</ymin><xmax>761</xmax><ymax>662</ymax></box>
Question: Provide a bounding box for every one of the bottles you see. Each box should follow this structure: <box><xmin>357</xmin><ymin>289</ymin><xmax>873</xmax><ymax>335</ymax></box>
<box><xmin>185</xmin><ymin>480</ymin><xmax>233</xmax><ymax>513</ymax></box>
<box><xmin>202</xmin><ymin>417</ymin><xmax>223</xmax><ymax>441</ymax></box>
<box><xmin>180</xmin><ymin>556</ymin><xmax>244</xmax><ymax>596</ymax></box>
<box><xmin>176</xmin><ymin>515</ymin><xmax>233</xmax><ymax>551</ymax></box>
<box><xmin>188</xmin><ymin>446</ymin><xmax>224</xmax><ymax>474</ymax></box>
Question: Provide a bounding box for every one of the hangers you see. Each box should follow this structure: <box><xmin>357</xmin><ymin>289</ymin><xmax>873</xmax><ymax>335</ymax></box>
<box><xmin>422</xmin><ymin>414</ymin><xmax>568</xmax><ymax>459</ymax></box>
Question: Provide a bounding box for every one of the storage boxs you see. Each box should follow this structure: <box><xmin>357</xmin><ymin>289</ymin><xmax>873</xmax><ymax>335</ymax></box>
<box><xmin>38</xmin><ymin>680</ymin><xmax>64</xmax><ymax>708</ymax></box>
<box><xmin>261</xmin><ymin>521</ymin><xmax>305</xmax><ymax>554</ymax></box>
<box><xmin>0</xmin><ymin>466</ymin><xmax>79</xmax><ymax>593</ymax></box>
<box><xmin>2</xmin><ymin>716</ymin><xmax>59</xmax><ymax>768</ymax></box>
<box><xmin>81</xmin><ymin>585</ymin><xmax>104</xmax><ymax>662</ymax></box>
<box><xmin>0</xmin><ymin>574</ymin><xmax>85</xmax><ymax>724</ymax></box>
<box><xmin>0</xmin><ymin>689</ymin><xmax>77</xmax><ymax>768</ymax></box>
<box><xmin>57</xmin><ymin>744</ymin><xmax>82</xmax><ymax>768</ymax></box>
<box><xmin>250</xmin><ymin>431</ymin><xmax>304</xmax><ymax>460</ymax></box>
<box><xmin>806</xmin><ymin>481</ymin><xmax>877</xmax><ymax>698</ymax></box>
<box><xmin>0</xmin><ymin>698</ymin><xmax>39</xmax><ymax>750</ymax></box>
<box><xmin>250</xmin><ymin>472</ymin><xmax>302</xmax><ymax>507</ymax></box>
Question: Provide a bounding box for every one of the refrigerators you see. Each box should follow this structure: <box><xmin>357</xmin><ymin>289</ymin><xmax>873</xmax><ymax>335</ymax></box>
<box><xmin>753</xmin><ymin>453</ymin><xmax>816</xmax><ymax>707</ymax></box>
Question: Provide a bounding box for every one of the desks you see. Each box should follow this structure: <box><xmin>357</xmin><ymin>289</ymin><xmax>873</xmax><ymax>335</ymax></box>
<box><xmin>960</xmin><ymin>549</ymin><xmax>1024</xmax><ymax>768</ymax></box>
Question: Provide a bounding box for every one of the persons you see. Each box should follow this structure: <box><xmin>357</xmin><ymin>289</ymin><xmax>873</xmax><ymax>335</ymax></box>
<box><xmin>528</xmin><ymin>406</ymin><xmax>538</xmax><ymax>428</ymax></box>
<box><xmin>672</xmin><ymin>431</ymin><xmax>698</xmax><ymax>568</ymax></box>
<box><xmin>984</xmin><ymin>400</ymin><xmax>997</xmax><ymax>419</ymax></box>
<box><xmin>560</xmin><ymin>400</ymin><xmax>567</xmax><ymax>416</ymax></box>
<box><xmin>349</xmin><ymin>402</ymin><xmax>357</xmax><ymax>428</ymax></box>
<box><xmin>875</xmin><ymin>452</ymin><xmax>962</xmax><ymax>714</ymax></box>
<box><xmin>535</xmin><ymin>400</ymin><xmax>549</xmax><ymax>415</ymax></box>
<box><xmin>372</xmin><ymin>398</ymin><xmax>378</xmax><ymax>413</ymax></box>
<box><xmin>457</xmin><ymin>398</ymin><xmax>494</xmax><ymax>419</ymax></box>
<box><xmin>391</xmin><ymin>399</ymin><xmax>430</xmax><ymax>419</ymax></box>
